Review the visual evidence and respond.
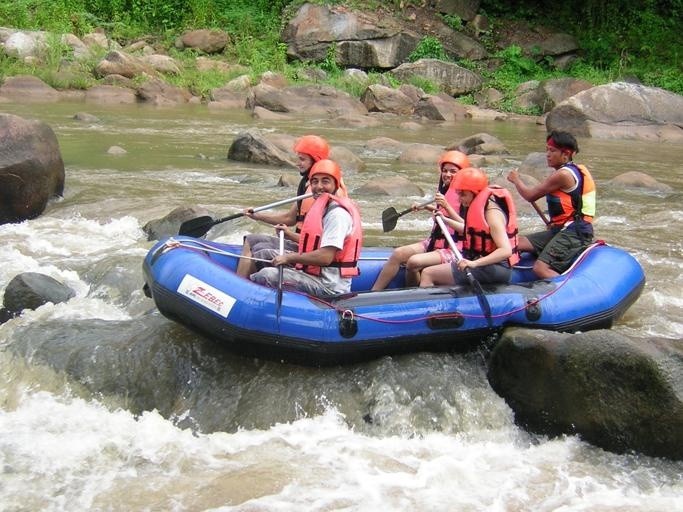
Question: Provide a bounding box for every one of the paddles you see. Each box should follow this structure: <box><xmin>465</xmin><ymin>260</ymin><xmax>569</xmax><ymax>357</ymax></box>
<box><xmin>178</xmin><ymin>192</ymin><xmax>313</xmax><ymax>238</ymax></box>
<box><xmin>382</xmin><ymin>191</ymin><xmax>440</xmax><ymax>232</ymax></box>
<box><xmin>435</xmin><ymin>211</ymin><xmax>492</xmax><ymax>327</ymax></box>
<box><xmin>275</xmin><ymin>222</ymin><xmax>285</xmax><ymax>335</ymax></box>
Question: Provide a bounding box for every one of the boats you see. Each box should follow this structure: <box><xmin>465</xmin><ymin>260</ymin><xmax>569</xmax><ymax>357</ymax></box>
<box><xmin>133</xmin><ymin>228</ymin><xmax>649</xmax><ymax>373</ymax></box>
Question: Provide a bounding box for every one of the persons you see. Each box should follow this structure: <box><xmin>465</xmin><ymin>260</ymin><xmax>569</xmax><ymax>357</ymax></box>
<box><xmin>371</xmin><ymin>151</ymin><xmax>470</xmax><ymax>292</ymax></box>
<box><xmin>418</xmin><ymin>167</ymin><xmax>520</xmax><ymax>288</ymax></box>
<box><xmin>508</xmin><ymin>130</ymin><xmax>596</xmax><ymax>279</ymax></box>
<box><xmin>236</xmin><ymin>134</ymin><xmax>348</xmax><ymax>281</ymax></box>
<box><xmin>248</xmin><ymin>159</ymin><xmax>362</xmax><ymax>301</ymax></box>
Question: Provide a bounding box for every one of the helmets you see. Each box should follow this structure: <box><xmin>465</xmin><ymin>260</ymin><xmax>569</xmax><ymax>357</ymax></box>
<box><xmin>294</xmin><ymin>135</ymin><xmax>329</xmax><ymax>160</ymax></box>
<box><xmin>439</xmin><ymin>151</ymin><xmax>470</xmax><ymax>169</ymax></box>
<box><xmin>309</xmin><ymin>160</ymin><xmax>341</xmax><ymax>187</ymax></box>
<box><xmin>453</xmin><ymin>169</ymin><xmax>488</xmax><ymax>194</ymax></box>
